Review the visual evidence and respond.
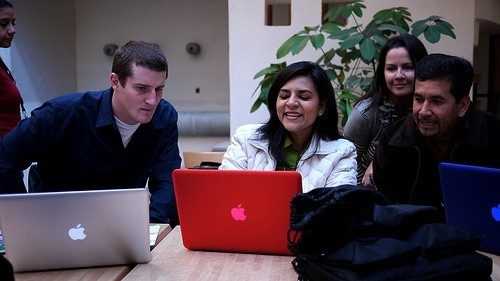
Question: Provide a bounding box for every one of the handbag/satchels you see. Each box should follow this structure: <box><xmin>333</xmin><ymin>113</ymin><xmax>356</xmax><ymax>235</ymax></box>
<box><xmin>287</xmin><ymin>184</ymin><xmax>439</xmax><ymax>256</ymax></box>
<box><xmin>291</xmin><ymin>220</ymin><xmax>494</xmax><ymax>281</ymax></box>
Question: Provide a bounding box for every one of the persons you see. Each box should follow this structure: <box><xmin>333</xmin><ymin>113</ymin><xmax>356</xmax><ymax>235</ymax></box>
<box><xmin>372</xmin><ymin>53</ymin><xmax>499</xmax><ymax>224</ymax></box>
<box><xmin>0</xmin><ymin>40</ymin><xmax>183</xmax><ymax>228</ymax></box>
<box><xmin>343</xmin><ymin>34</ymin><xmax>430</xmax><ymax>192</ymax></box>
<box><xmin>0</xmin><ymin>1</ymin><xmax>28</xmax><ymax>194</ymax></box>
<box><xmin>218</xmin><ymin>61</ymin><xmax>357</xmax><ymax>194</ymax></box>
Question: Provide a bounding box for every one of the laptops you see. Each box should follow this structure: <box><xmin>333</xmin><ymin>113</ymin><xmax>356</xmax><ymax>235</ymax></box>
<box><xmin>0</xmin><ymin>188</ymin><xmax>151</xmax><ymax>273</ymax></box>
<box><xmin>439</xmin><ymin>163</ymin><xmax>500</xmax><ymax>257</ymax></box>
<box><xmin>173</xmin><ymin>169</ymin><xmax>303</xmax><ymax>256</ymax></box>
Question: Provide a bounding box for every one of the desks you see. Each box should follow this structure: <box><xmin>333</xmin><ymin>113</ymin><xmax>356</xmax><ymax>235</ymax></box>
<box><xmin>0</xmin><ymin>224</ymin><xmax>500</xmax><ymax>281</ymax></box>
